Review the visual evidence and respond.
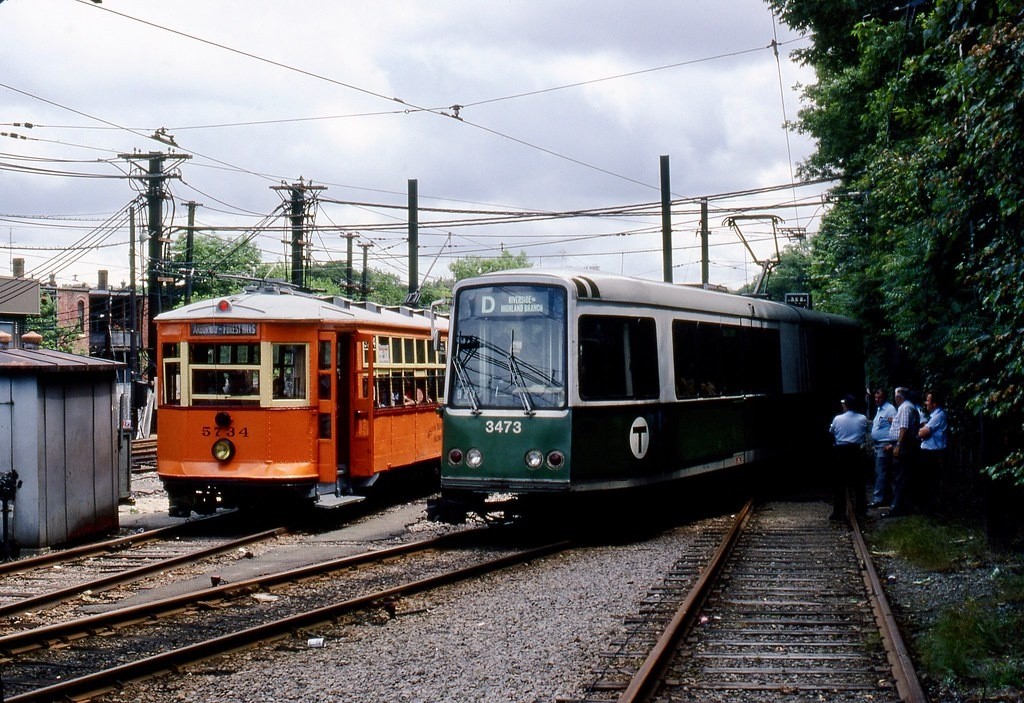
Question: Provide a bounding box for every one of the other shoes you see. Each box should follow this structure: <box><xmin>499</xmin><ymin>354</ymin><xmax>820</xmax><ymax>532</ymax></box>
<box><xmin>868</xmin><ymin>500</ymin><xmax>882</xmax><ymax>509</ymax></box>
<box><xmin>879</xmin><ymin>509</ymin><xmax>895</xmax><ymax>520</ymax></box>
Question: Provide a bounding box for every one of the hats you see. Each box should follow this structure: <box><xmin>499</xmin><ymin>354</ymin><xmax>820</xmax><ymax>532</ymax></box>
<box><xmin>894</xmin><ymin>387</ymin><xmax>905</xmax><ymax>400</ymax></box>
<box><xmin>841</xmin><ymin>395</ymin><xmax>855</xmax><ymax>404</ymax></box>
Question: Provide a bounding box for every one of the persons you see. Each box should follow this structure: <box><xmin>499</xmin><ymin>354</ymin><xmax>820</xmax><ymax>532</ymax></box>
<box><xmin>192</xmin><ymin>370</ymin><xmax>259</xmax><ymax>405</ymax></box>
<box><xmin>373</xmin><ymin>375</ymin><xmax>436</xmax><ymax>408</ymax></box>
<box><xmin>273</xmin><ymin>375</ymin><xmax>294</xmax><ymax>398</ymax></box>
<box><xmin>829</xmin><ymin>396</ymin><xmax>868</xmax><ymax>522</ymax></box>
<box><xmin>869</xmin><ymin>386</ymin><xmax>947</xmax><ymax>517</ymax></box>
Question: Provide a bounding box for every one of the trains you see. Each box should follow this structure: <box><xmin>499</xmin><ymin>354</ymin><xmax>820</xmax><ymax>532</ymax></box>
<box><xmin>427</xmin><ymin>270</ymin><xmax>873</xmax><ymax>529</ymax></box>
<box><xmin>153</xmin><ymin>275</ymin><xmax>450</xmax><ymax>518</ymax></box>
